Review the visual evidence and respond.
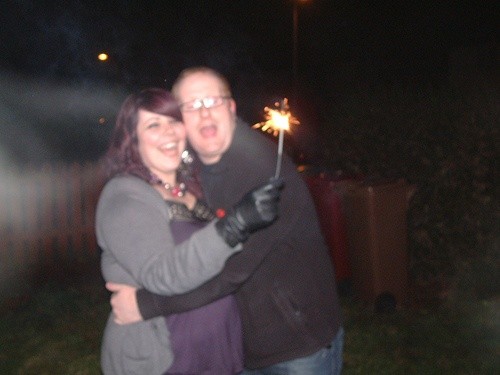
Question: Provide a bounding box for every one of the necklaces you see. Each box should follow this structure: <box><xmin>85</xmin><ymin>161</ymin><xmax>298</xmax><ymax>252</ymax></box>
<box><xmin>143</xmin><ymin>168</ymin><xmax>187</xmax><ymax>199</ymax></box>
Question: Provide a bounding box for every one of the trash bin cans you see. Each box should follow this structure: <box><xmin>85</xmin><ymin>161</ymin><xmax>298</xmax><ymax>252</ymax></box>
<box><xmin>340</xmin><ymin>174</ymin><xmax>416</xmax><ymax>312</ymax></box>
<box><xmin>301</xmin><ymin>168</ymin><xmax>355</xmax><ymax>288</ymax></box>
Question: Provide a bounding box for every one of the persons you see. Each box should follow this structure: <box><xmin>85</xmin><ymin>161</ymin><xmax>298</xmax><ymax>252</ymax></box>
<box><xmin>94</xmin><ymin>92</ymin><xmax>286</xmax><ymax>375</ymax></box>
<box><xmin>105</xmin><ymin>66</ymin><xmax>348</xmax><ymax>375</ymax></box>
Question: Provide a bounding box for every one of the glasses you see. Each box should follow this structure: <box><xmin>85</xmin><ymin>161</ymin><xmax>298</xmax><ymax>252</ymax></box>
<box><xmin>177</xmin><ymin>96</ymin><xmax>233</xmax><ymax>112</ymax></box>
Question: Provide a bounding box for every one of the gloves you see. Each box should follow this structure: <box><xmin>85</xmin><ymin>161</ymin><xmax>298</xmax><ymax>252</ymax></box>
<box><xmin>216</xmin><ymin>177</ymin><xmax>285</xmax><ymax>250</ymax></box>
